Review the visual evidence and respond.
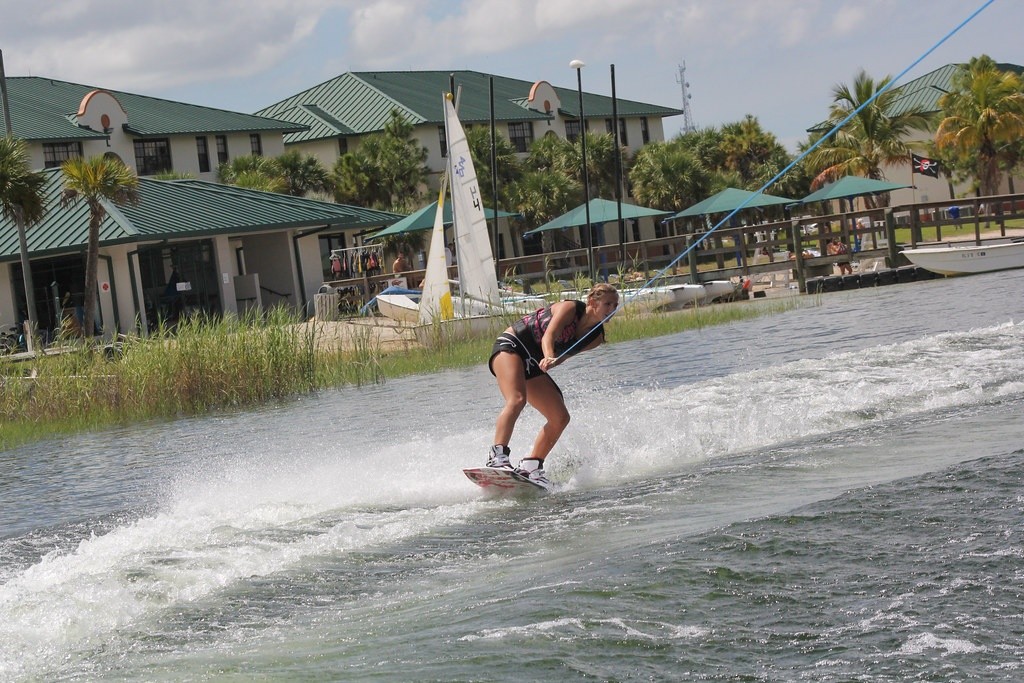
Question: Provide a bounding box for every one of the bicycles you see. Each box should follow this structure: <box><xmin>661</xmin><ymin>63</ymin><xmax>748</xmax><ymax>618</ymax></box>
<box><xmin>0</xmin><ymin>310</ymin><xmax>28</xmax><ymax>356</ymax></box>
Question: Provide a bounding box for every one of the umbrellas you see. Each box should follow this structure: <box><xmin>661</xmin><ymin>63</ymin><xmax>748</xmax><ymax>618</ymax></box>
<box><xmin>522</xmin><ymin>198</ymin><xmax>676</xmax><ymax>283</ymax></box>
<box><xmin>661</xmin><ymin>188</ymin><xmax>802</xmax><ymax>282</ymax></box>
<box><xmin>364</xmin><ymin>198</ymin><xmax>521</xmax><ymax>248</ymax></box>
<box><xmin>785</xmin><ymin>175</ymin><xmax>917</xmax><ymax>252</ymax></box>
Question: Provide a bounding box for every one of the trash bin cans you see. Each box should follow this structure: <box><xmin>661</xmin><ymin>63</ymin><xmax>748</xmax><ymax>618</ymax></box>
<box><xmin>314</xmin><ymin>284</ymin><xmax>340</xmax><ymax>322</ymax></box>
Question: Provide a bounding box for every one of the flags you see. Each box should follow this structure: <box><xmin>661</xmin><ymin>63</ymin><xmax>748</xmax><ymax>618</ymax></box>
<box><xmin>912</xmin><ymin>153</ymin><xmax>940</xmax><ymax>179</ymax></box>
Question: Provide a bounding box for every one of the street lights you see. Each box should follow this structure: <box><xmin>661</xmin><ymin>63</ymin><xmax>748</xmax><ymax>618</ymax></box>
<box><xmin>568</xmin><ymin>59</ymin><xmax>597</xmax><ymax>286</ymax></box>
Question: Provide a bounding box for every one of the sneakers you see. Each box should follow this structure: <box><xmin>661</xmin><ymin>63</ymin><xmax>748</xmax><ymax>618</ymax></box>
<box><xmin>518</xmin><ymin>457</ymin><xmax>549</xmax><ymax>486</ymax></box>
<box><xmin>486</xmin><ymin>445</ymin><xmax>530</xmax><ymax>478</ymax></box>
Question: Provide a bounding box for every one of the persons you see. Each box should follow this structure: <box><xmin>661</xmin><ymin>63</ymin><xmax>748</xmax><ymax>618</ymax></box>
<box><xmin>393</xmin><ymin>251</ymin><xmax>411</xmax><ymax>289</ymax></box>
<box><xmin>978</xmin><ymin>204</ymin><xmax>987</xmax><ymax>213</ymax></box>
<box><xmin>485</xmin><ymin>283</ymin><xmax>620</xmax><ymax>485</ymax></box>
<box><xmin>446</xmin><ymin>238</ymin><xmax>458</xmax><ymax>296</ymax></box>
<box><xmin>419</xmin><ymin>279</ymin><xmax>425</xmax><ymax>287</ymax></box>
<box><xmin>857</xmin><ymin>219</ymin><xmax>863</xmax><ymax>249</ymax></box>
<box><xmin>789</xmin><ymin>250</ymin><xmax>796</xmax><ymax>260</ymax></box>
<box><xmin>949</xmin><ymin>206</ymin><xmax>962</xmax><ymax>229</ymax></box>
<box><xmin>831</xmin><ymin>239</ymin><xmax>852</xmax><ymax>275</ymax></box>
<box><xmin>381</xmin><ymin>280</ymin><xmax>399</xmax><ymax>292</ymax></box>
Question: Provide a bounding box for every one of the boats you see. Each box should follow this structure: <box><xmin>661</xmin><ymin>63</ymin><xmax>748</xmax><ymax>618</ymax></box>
<box><xmin>375</xmin><ymin>84</ymin><xmax>754</xmax><ymax>348</ymax></box>
<box><xmin>358</xmin><ymin>287</ymin><xmax>422</xmax><ymax>316</ymax></box>
<box><xmin>898</xmin><ymin>242</ymin><xmax>1024</xmax><ymax>278</ymax></box>
